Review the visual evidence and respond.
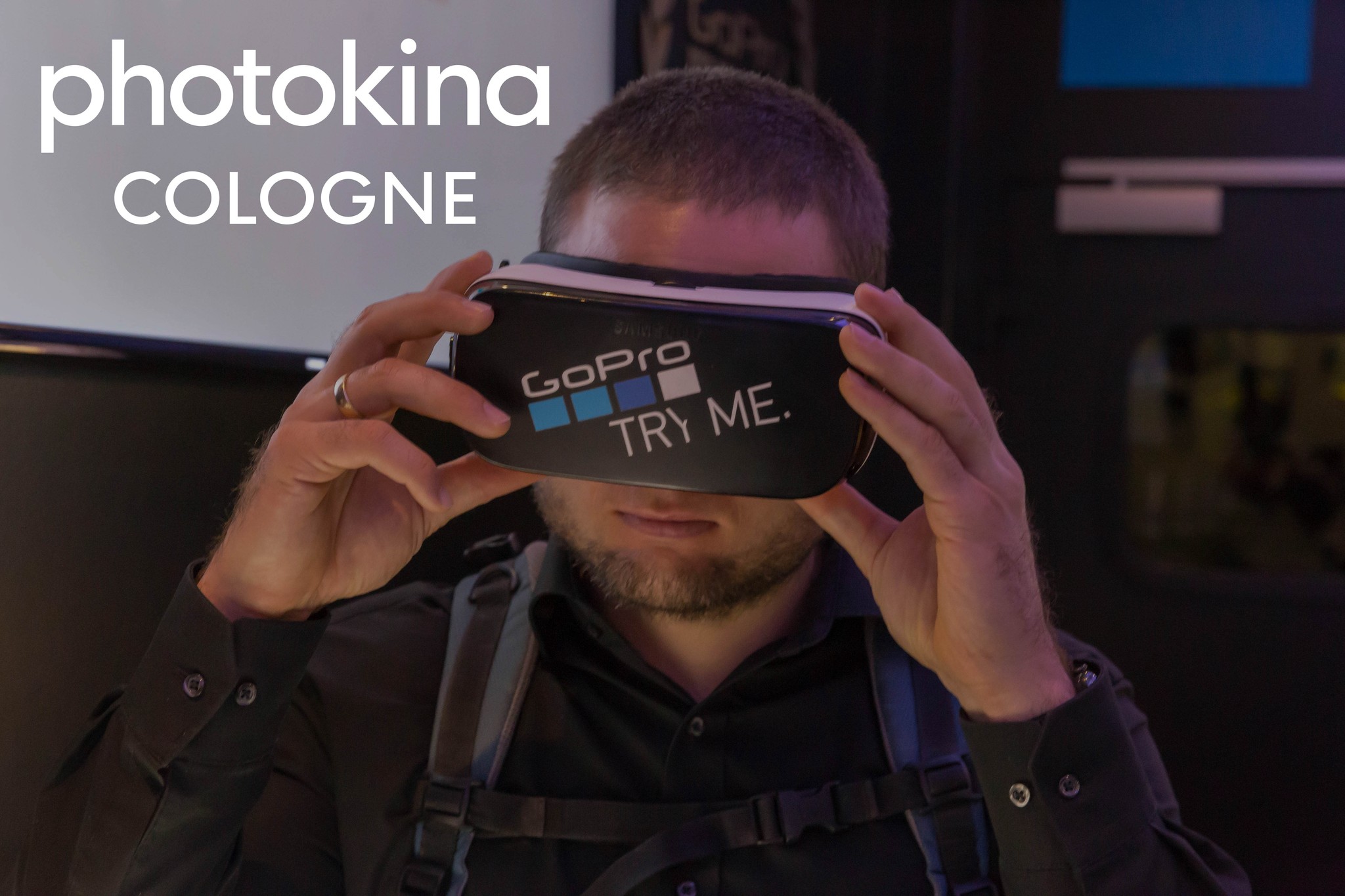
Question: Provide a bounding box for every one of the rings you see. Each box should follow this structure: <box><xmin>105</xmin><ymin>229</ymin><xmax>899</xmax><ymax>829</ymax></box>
<box><xmin>332</xmin><ymin>370</ymin><xmax>370</xmax><ymax>421</ymax></box>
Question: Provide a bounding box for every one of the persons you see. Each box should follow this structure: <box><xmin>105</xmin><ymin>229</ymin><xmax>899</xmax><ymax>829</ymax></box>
<box><xmin>27</xmin><ymin>66</ymin><xmax>1249</xmax><ymax>896</ymax></box>
